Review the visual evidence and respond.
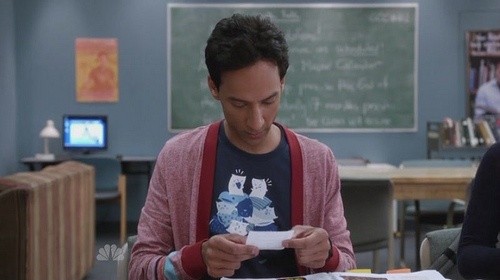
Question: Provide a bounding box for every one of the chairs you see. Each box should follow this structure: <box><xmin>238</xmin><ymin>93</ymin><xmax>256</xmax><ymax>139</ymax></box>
<box><xmin>71</xmin><ymin>156</ymin><xmax>128</xmax><ymax>246</ymax></box>
<box><xmin>340</xmin><ymin>158</ymin><xmax>480</xmax><ymax>280</ymax></box>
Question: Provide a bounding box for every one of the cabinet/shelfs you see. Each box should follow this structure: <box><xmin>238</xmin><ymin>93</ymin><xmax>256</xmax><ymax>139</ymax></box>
<box><xmin>465</xmin><ymin>28</ymin><xmax>500</xmax><ymax>120</ymax></box>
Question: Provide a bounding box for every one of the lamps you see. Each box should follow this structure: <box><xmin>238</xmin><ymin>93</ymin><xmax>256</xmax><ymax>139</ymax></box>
<box><xmin>35</xmin><ymin>119</ymin><xmax>60</xmax><ymax>160</ymax></box>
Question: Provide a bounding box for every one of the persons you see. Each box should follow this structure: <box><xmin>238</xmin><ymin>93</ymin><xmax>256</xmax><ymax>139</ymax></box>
<box><xmin>457</xmin><ymin>63</ymin><xmax>500</xmax><ymax>280</ymax></box>
<box><xmin>126</xmin><ymin>12</ymin><xmax>356</xmax><ymax>280</ymax></box>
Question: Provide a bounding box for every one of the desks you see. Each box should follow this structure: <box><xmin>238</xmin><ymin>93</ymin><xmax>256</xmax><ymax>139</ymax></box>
<box><xmin>386</xmin><ymin>166</ymin><xmax>476</xmax><ymax>271</ymax></box>
<box><xmin>20</xmin><ymin>155</ymin><xmax>157</xmax><ymax>188</ymax></box>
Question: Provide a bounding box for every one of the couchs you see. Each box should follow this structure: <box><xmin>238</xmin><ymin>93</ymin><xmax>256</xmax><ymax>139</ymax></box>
<box><xmin>0</xmin><ymin>160</ymin><xmax>96</xmax><ymax>280</ymax></box>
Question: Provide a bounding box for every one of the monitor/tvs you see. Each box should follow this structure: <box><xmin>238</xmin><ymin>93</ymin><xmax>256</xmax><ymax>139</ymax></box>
<box><xmin>62</xmin><ymin>114</ymin><xmax>107</xmax><ymax>150</ymax></box>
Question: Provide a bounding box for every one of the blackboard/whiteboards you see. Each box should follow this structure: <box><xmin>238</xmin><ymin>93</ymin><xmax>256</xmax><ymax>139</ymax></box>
<box><xmin>166</xmin><ymin>3</ymin><xmax>419</xmax><ymax>134</ymax></box>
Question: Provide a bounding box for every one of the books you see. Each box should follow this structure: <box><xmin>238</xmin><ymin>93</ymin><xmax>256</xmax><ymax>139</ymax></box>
<box><xmin>427</xmin><ymin>116</ymin><xmax>497</xmax><ymax>149</ymax></box>
